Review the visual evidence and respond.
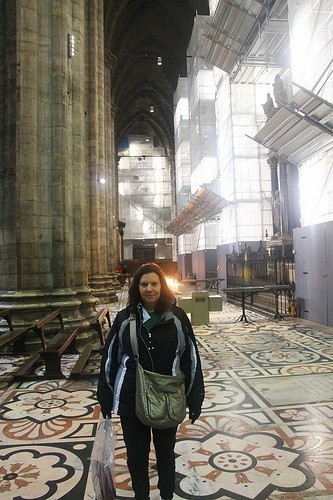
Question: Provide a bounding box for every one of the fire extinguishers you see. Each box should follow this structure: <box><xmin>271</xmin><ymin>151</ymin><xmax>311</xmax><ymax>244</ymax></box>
<box><xmin>288</xmin><ymin>300</ymin><xmax>296</xmax><ymax>318</ymax></box>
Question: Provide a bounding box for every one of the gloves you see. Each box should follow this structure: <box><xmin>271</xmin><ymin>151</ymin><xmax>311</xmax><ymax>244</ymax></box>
<box><xmin>188</xmin><ymin>403</ymin><xmax>201</xmax><ymax>424</ymax></box>
<box><xmin>101</xmin><ymin>403</ymin><xmax>113</xmax><ymax>419</ymax></box>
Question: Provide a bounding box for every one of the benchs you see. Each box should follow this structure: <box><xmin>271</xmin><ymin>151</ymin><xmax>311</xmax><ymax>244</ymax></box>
<box><xmin>0</xmin><ymin>305</ymin><xmax>112</xmax><ymax>380</ymax></box>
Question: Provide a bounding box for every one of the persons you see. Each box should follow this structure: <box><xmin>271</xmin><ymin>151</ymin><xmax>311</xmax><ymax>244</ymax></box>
<box><xmin>96</xmin><ymin>261</ymin><xmax>206</xmax><ymax>500</ymax></box>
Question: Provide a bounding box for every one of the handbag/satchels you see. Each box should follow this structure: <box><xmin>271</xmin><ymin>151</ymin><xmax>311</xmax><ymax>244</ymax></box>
<box><xmin>136</xmin><ymin>364</ymin><xmax>186</xmax><ymax>429</ymax></box>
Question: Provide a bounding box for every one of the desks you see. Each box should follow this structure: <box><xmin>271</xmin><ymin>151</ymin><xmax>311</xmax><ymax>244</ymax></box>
<box><xmin>261</xmin><ymin>285</ymin><xmax>293</xmax><ymax>321</ymax></box>
<box><xmin>182</xmin><ymin>278</ymin><xmax>225</xmax><ymax>294</ymax></box>
<box><xmin>223</xmin><ymin>287</ymin><xmax>265</xmax><ymax>323</ymax></box>
